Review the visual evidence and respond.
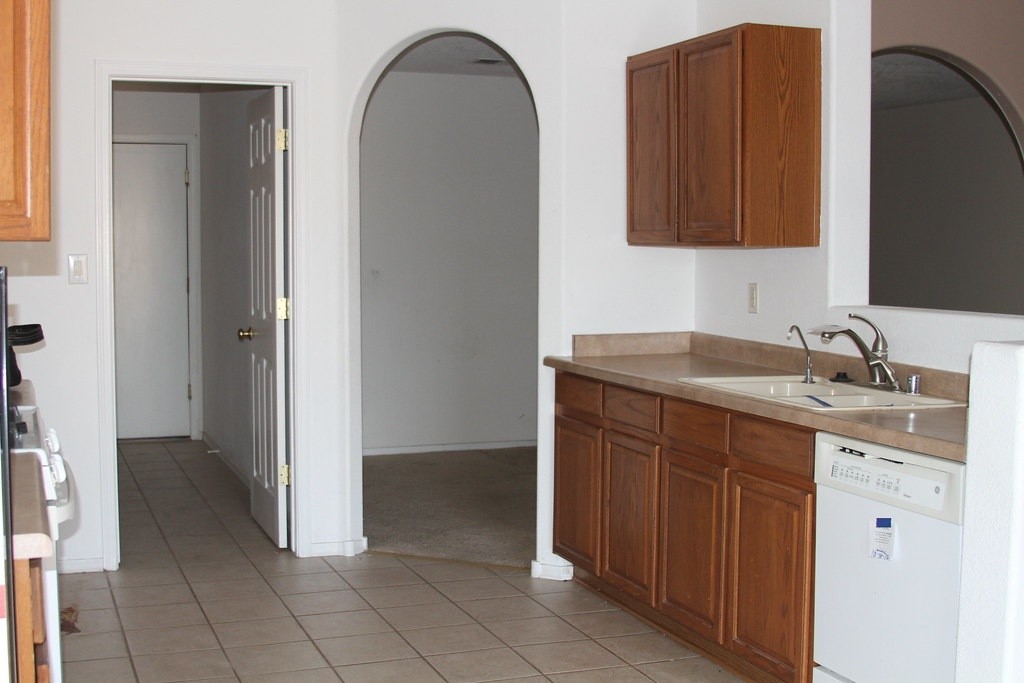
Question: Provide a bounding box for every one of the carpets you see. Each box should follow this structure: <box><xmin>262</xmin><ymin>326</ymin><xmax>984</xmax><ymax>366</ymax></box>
<box><xmin>363</xmin><ymin>444</ymin><xmax>537</xmax><ymax>566</ymax></box>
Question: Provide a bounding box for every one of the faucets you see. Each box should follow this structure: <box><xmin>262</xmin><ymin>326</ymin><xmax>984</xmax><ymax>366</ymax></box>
<box><xmin>819</xmin><ymin>313</ymin><xmax>890</xmax><ymax>385</ymax></box>
<box><xmin>786</xmin><ymin>323</ymin><xmax>815</xmax><ymax>384</ymax></box>
<box><xmin>868</xmin><ymin>357</ymin><xmax>902</xmax><ymax>391</ymax></box>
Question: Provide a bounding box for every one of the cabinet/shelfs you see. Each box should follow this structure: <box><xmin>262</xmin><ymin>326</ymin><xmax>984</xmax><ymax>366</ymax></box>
<box><xmin>627</xmin><ymin>22</ymin><xmax>822</xmax><ymax>247</ymax></box>
<box><xmin>1</xmin><ymin>1</ymin><xmax>51</xmax><ymax>241</ymax></box>
<box><xmin>553</xmin><ymin>369</ymin><xmax>818</xmax><ymax>683</ymax></box>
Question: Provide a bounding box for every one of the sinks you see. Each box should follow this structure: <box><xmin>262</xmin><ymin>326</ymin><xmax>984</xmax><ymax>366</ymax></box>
<box><xmin>710</xmin><ymin>378</ymin><xmax>863</xmax><ymax>398</ymax></box>
<box><xmin>776</xmin><ymin>391</ymin><xmax>953</xmax><ymax>410</ymax></box>
